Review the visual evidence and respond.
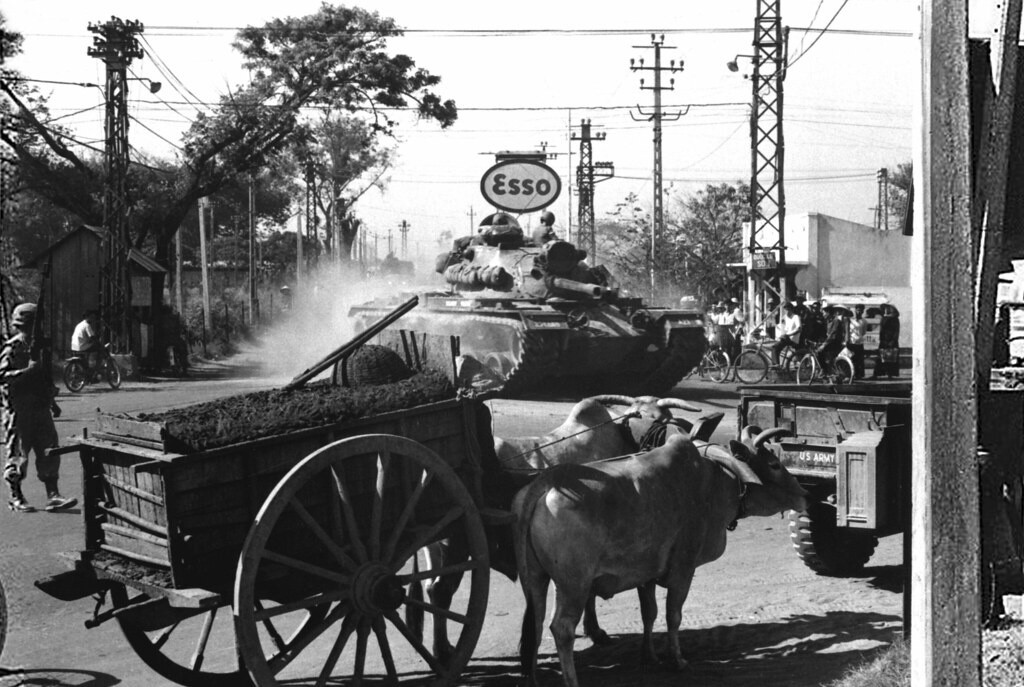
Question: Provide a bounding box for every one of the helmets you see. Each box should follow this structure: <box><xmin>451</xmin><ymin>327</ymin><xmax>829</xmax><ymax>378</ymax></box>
<box><xmin>11</xmin><ymin>303</ymin><xmax>38</xmax><ymax>326</ymax></box>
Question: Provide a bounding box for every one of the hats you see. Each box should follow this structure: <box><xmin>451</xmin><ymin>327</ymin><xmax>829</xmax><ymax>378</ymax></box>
<box><xmin>767</xmin><ymin>297</ymin><xmax>774</xmax><ymax>303</ymax></box>
<box><xmin>832</xmin><ymin>304</ymin><xmax>854</xmax><ymax>318</ymax></box>
<box><xmin>731</xmin><ymin>298</ymin><xmax>738</xmax><ymax>303</ymax></box>
<box><xmin>716</xmin><ymin>302</ymin><xmax>725</xmax><ymax>307</ymax></box>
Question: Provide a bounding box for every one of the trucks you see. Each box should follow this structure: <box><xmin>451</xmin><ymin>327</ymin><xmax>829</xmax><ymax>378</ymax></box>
<box><xmin>735</xmin><ymin>300</ymin><xmax>1024</xmax><ymax>577</ymax></box>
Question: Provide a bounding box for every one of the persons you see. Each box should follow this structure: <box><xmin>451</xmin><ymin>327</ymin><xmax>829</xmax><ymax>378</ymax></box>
<box><xmin>766</xmin><ymin>296</ymin><xmax>867</xmax><ymax>377</ymax></box>
<box><xmin>71</xmin><ymin>309</ymin><xmax>110</xmax><ymax>388</ymax></box>
<box><xmin>765</xmin><ymin>302</ymin><xmax>778</xmax><ymax>340</ymax></box>
<box><xmin>0</xmin><ymin>303</ymin><xmax>78</xmax><ymax>513</ymax></box>
<box><xmin>994</xmin><ymin>307</ymin><xmax>1011</xmax><ymax>367</ymax></box>
<box><xmin>533</xmin><ymin>212</ymin><xmax>557</xmax><ymax>247</ymax></box>
<box><xmin>867</xmin><ymin>305</ymin><xmax>900</xmax><ymax>381</ymax></box>
<box><xmin>707</xmin><ymin>298</ymin><xmax>745</xmax><ymax>368</ymax></box>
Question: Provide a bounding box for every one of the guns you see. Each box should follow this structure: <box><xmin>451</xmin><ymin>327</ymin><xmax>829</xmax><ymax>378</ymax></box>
<box><xmin>27</xmin><ymin>242</ymin><xmax>58</xmax><ymax>363</ymax></box>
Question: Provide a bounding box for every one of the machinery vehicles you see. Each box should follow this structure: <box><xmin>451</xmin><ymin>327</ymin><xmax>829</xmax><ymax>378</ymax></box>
<box><xmin>346</xmin><ymin>212</ymin><xmax>708</xmax><ymax>399</ymax></box>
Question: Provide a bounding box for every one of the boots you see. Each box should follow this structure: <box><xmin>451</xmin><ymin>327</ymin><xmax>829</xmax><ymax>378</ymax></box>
<box><xmin>8</xmin><ymin>483</ymin><xmax>34</xmax><ymax>512</ymax></box>
<box><xmin>45</xmin><ymin>479</ymin><xmax>77</xmax><ymax>510</ymax></box>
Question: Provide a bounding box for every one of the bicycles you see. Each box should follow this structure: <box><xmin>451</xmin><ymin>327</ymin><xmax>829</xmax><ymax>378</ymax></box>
<box><xmin>63</xmin><ymin>338</ymin><xmax>123</xmax><ymax>392</ymax></box>
<box><xmin>682</xmin><ymin>327</ymin><xmax>855</xmax><ymax>384</ymax></box>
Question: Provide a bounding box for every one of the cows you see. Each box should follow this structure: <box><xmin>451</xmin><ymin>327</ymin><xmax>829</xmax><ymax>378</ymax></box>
<box><xmin>424</xmin><ymin>393</ymin><xmax>809</xmax><ymax>687</ymax></box>
